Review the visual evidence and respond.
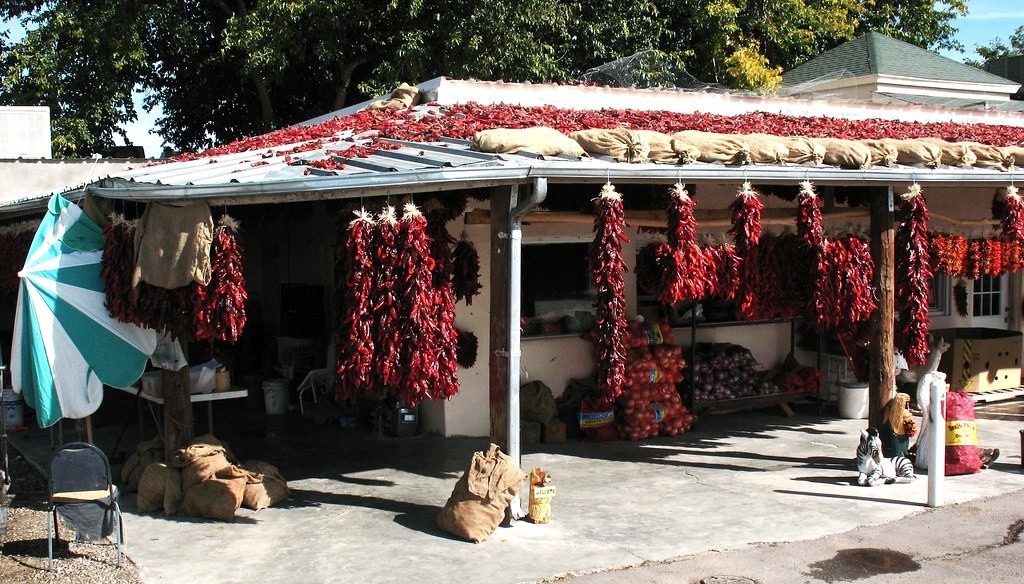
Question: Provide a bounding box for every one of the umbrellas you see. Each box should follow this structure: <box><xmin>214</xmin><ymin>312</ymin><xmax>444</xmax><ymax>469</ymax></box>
<box><xmin>10</xmin><ymin>193</ymin><xmax>158</xmax><ymax>445</ymax></box>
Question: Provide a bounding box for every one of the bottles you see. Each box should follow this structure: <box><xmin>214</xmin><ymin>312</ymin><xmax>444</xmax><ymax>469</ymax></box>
<box><xmin>215</xmin><ymin>366</ymin><xmax>231</xmax><ymax>392</ymax></box>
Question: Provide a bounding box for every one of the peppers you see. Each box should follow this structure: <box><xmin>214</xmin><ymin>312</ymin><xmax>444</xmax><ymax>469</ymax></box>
<box><xmin>102</xmin><ymin>103</ymin><xmax>1024</xmax><ymax>406</ymax></box>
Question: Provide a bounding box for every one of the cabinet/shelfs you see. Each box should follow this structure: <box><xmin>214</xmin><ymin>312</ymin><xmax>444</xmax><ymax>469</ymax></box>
<box><xmin>670</xmin><ymin>315</ymin><xmax>824</xmax><ymax>431</ymax></box>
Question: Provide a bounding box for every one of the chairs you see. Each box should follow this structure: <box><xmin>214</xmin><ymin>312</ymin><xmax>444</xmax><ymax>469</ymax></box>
<box><xmin>48</xmin><ymin>442</ymin><xmax>123</xmax><ymax>569</ymax></box>
<box><xmin>275</xmin><ymin>336</ymin><xmax>336</xmax><ymax>416</ymax></box>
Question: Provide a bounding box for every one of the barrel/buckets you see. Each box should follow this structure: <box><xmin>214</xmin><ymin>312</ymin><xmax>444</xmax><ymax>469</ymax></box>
<box><xmin>262</xmin><ymin>379</ymin><xmax>289</xmax><ymax>414</ymax></box>
<box><xmin>0</xmin><ymin>389</ymin><xmax>24</xmax><ymax>426</ymax></box>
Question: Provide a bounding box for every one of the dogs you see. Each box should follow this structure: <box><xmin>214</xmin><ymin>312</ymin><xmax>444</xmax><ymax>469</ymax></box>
<box><xmin>899</xmin><ymin>333</ymin><xmax>951</xmax><ymax>386</ymax></box>
<box><xmin>529</xmin><ymin>466</ymin><xmax>556</xmax><ymax>524</ymax></box>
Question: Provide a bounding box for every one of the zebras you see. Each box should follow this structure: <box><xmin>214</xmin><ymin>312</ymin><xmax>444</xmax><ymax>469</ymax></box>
<box><xmin>857</xmin><ymin>430</ymin><xmax>915</xmax><ymax>487</ymax></box>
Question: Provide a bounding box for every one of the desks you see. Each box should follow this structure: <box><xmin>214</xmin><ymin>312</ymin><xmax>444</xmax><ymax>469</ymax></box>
<box><xmin>103</xmin><ymin>383</ymin><xmax>248</xmax><ymax>436</ymax></box>
